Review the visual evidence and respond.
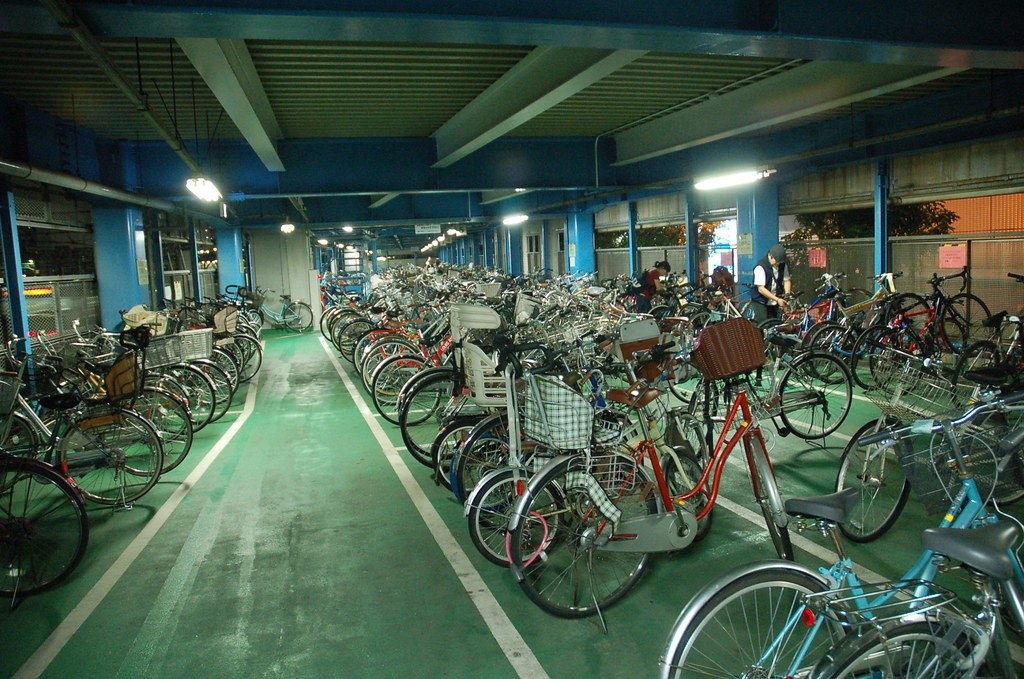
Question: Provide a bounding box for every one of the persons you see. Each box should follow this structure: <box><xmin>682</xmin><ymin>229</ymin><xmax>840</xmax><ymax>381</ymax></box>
<box><xmin>626</xmin><ymin>260</ymin><xmax>670</xmax><ymax>314</ymax></box>
<box><xmin>751</xmin><ymin>244</ymin><xmax>791</xmax><ymax>330</ymax></box>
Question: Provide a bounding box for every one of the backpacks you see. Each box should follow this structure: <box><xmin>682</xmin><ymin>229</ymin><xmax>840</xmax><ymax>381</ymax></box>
<box><xmin>712</xmin><ymin>265</ymin><xmax>734</xmax><ymax>289</ymax></box>
<box><xmin>625</xmin><ymin>269</ymin><xmax>650</xmax><ymax>296</ymax></box>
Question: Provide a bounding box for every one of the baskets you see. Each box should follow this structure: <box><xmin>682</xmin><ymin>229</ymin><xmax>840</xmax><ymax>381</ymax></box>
<box><xmin>480</xmin><ymin>282</ymin><xmax>502</xmax><ymax>298</ymax></box>
<box><xmin>891</xmin><ymin>397</ymin><xmax>1022</xmax><ymax>515</ymax></box>
<box><xmin>864</xmin><ymin>347</ymin><xmax>977</xmax><ymax>423</ymax></box>
<box><xmin>689</xmin><ymin>318</ymin><xmax>767</xmax><ymax>380</ymax></box>
<box><xmin>0</xmin><ymin>292</ymin><xmax>265</xmax><ymax>423</ymax></box>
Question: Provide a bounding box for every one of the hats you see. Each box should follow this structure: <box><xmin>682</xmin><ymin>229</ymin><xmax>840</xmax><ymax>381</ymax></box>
<box><xmin>770</xmin><ymin>244</ymin><xmax>787</xmax><ymax>263</ymax></box>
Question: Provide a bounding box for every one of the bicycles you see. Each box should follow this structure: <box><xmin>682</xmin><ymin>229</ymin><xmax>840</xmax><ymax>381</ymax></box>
<box><xmin>0</xmin><ymin>246</ymin><xmax>1024</xmax><ymax>679</ymax></box>
